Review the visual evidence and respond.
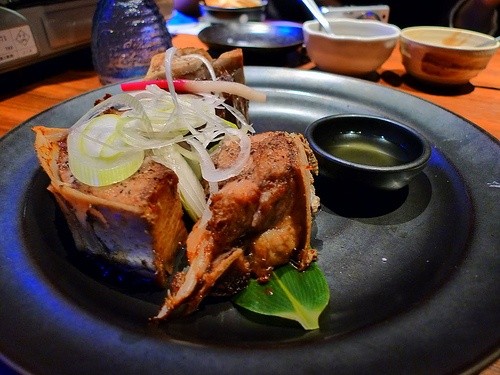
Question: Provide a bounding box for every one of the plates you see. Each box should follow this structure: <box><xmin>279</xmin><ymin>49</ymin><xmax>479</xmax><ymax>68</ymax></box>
<box><xmin>0</xmin><ymin>65</ymin><xmax>500</xmax><ymax>375</ymax></box>
<box><xmin>198</xmin><ymin>22</ymin><xmax>306</xmax><ymax>64</ymax></box>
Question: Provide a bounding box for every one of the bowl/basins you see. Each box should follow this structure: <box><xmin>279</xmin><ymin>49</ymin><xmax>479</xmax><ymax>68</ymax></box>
<box><xmin>305</xmin><ymin>114</ymin><xmax>432</xmax><ymax>192</ymax></box>
<box><xmin>302</xmin><ymin>18</ymin><xmax>402</xmax><ymax>75</ymax></box>
<box><xmin>400</xmin><ymin>26</ymin><xmax>499</xmax><ymax>84</ymax></box>
<box><xmin>322</xmin><ymin>5</ymin><xmax>388</xmax><ymax>23</ymax></box>
<box><xmin>198</xmin><ymin>0</ymin><xmax>268</xmax><ymax>23</ymax></box>
